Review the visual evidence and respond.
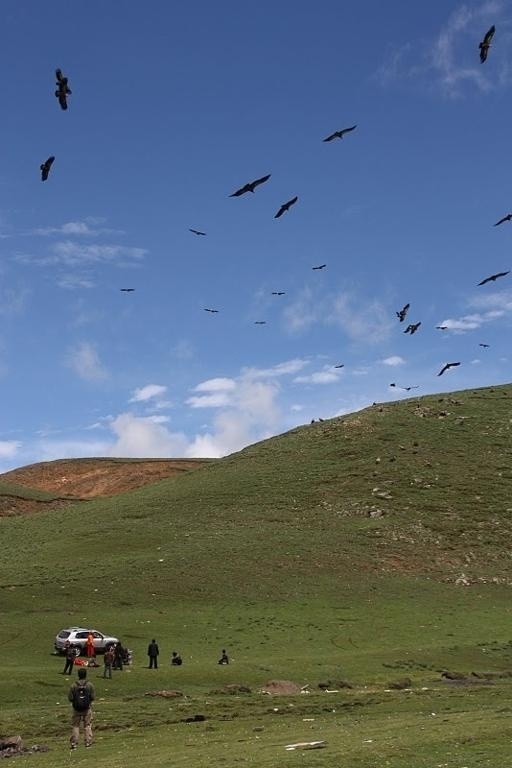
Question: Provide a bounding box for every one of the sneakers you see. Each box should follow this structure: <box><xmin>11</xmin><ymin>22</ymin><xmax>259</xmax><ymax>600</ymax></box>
<box><xmin>72</xmin><ymin>744</ymin><xmax>76</xmax><ymax>748</ymax></box>
<box><xmin>85</xmin><ymin>742</ymin><xmax>90</xmax><ymax>747</ymax></box>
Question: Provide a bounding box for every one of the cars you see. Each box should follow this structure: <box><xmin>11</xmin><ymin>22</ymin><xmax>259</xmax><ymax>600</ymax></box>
<box><xmin>54</xmin><ymin>627</ymin><xmax>119</xmax><ymax>657</ymax></box>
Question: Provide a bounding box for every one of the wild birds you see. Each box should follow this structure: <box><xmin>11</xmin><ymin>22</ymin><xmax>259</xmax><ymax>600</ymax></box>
<box><xmin>189</xmin><ymin>228</ymin><xmax>205</xmax><ymax>236</ymax></box>
<box><xmin>120</xmin><ymin>288</ymin><xmax>135</xmax><ymax>291</ymax></box>
<box><xmin>333</xmin><ymin>363</ymin><xmax>344</xmax><ymax>370</ymax></box>
<box><xmin>54</xmin><ymin>69</ymin><xmax>72</xmax><ymax>111</ymax></box>
<box><xmin>493</xmin><ymin>214</ymin><xmax>512</xmax><ymax>227</ymax></box>
<box><xmin>228</xmin><ymin>174</ymin><xmax>299</xmax><ymax>220</ymax></box>
<box><xmin>202</xmin><ymin>263</ymin><xmax>328</xmax><ymax>326</ymax></box>
<box><xmin>388</xmin><ymin>302</ymin><xmax>492</xmax><ymax>391</ymax></box>
<box><xmin>479</xmin><ymin>25</ymin><xmax>495</xmax><ymax>64</ymax></box>
<box><xmin>40</xmin><ymin>156</ymin><xmax>55</xmax><ymax>181</ymax></box>
<box><xmin>322</xmin><ymin>124</ymin><xmax>357</xmax><ymax>142</ymax></box>
<box><xmin>479</xmin><ymin>271</ymin><xmax>510</xmax><ymax>285</ymax></box>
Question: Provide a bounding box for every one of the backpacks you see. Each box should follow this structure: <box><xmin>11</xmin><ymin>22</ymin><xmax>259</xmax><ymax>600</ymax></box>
<box><xmin>73</xmin><ymin>682</ymin><xmax>91</xmax><ymax>711</ymax></box>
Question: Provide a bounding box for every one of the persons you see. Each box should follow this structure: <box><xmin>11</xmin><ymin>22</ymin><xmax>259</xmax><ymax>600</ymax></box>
<box><xmin>148</xmin><ymin>639</ymin><xmax>159</xmax><ymax>668</ymax></box>
<box><xmin>171</xmin><ymin>651</ymin><xmax>182</xmax><ymax>665</ymax></box>
<box><xmin>63</xmin><ymin>632</ymin><xmax>130</xmax><ymax>678</ymax></box>
<box><xmin>218</xmin><ymin>650</ymin><xmax>228</xmax><ymax>665</ymax></box>
<box><xmin>68</xmin><ymin>668</ymin><xmax>96</xmax><ymax>749</ymax></box>
